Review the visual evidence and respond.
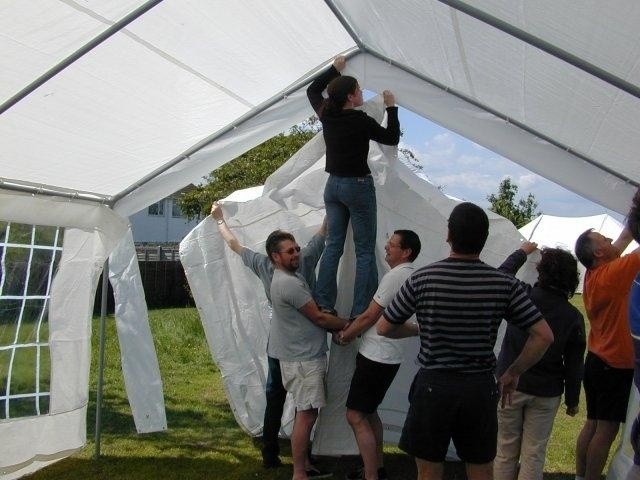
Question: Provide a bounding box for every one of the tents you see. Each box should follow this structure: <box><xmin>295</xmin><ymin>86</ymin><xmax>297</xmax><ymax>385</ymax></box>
<box><xmin>0</xmin><ymin>0</ymin><xmax>639</xmax><ymax>480</ymax></box>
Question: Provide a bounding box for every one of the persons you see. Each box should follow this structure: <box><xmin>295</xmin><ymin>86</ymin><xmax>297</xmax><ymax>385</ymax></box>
<box><xmin>306</xmin><ymin>57</ymin><xmax>402</xmax><ymax>316</ymax></box>
<box><xmin>627</xmin><ymin>188</ymin><xmax>640</xmax><ymax>479</ymax></box>
<box><xmin>206</xmin><ymin>200</ymin><xmax>328</xmax><ymax>470</ymax></box>
<box><xmin>574</xmin><ymin>224</ymin><xmax>639</xmax><ymax>480</ymax></box>
<box><xmin>266</xmin><ymin>231</ymin><xmax>352</xmax><ymax>480</ymax></box>
<box><xmin>493</xmin><ymin>239</ymin><xmax>586</xmax><ymax>479</ymax></box>
<box><xmin>328</xmin><ymin>230</ymin><xmax>421</xmax><ymax>480</ymax></box>
<box><xmin>376</xmin><ymin>203</ymin><xmax>555</xmax><ymax>480</ymax></box>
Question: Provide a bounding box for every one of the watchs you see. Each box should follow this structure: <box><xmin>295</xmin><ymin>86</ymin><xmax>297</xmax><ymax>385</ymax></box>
<box><xmin>217</xmin><ymin>219</ymin><xmax>225</xmax><ymax>225</ymax></box>
